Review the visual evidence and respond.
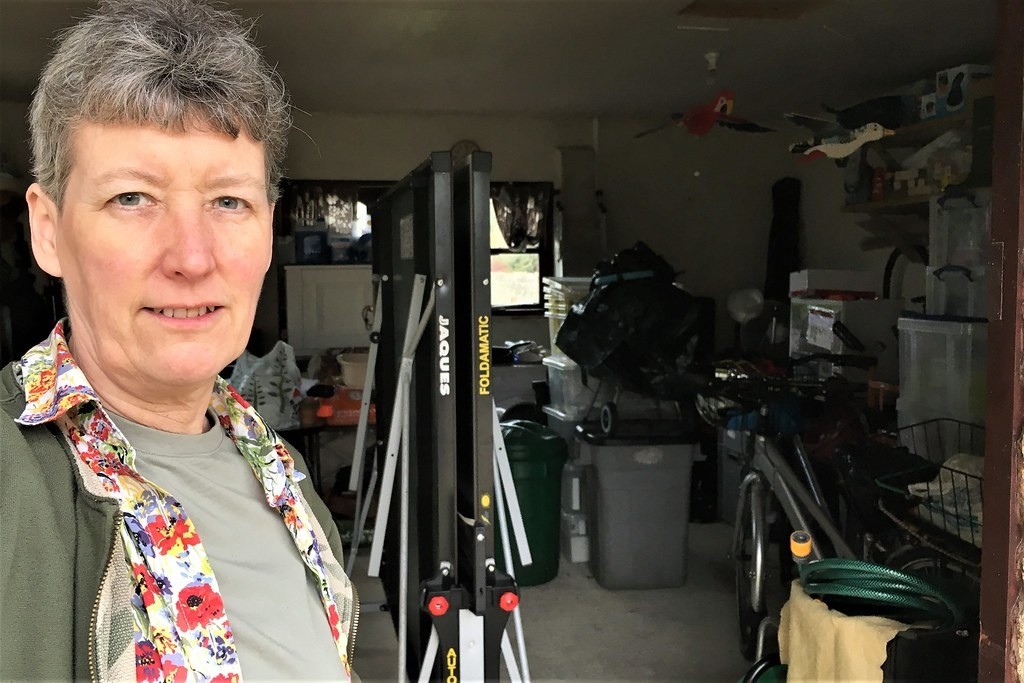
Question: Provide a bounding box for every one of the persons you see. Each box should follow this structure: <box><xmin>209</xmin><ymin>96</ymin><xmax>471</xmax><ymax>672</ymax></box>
<box><xmin>0</xmin><ymin>0</ymin><xmax>362</xmax><ymax>682</ymax></box>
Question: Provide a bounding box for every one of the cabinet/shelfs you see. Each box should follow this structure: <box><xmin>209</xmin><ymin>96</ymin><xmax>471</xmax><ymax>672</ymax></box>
<box><xmin>840</xmin><ymin>99</ymin><xmax>996</xmax><ymax>217</ymax></box>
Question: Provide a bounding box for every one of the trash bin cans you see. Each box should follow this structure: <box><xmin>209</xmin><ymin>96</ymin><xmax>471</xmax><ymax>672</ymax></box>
<box><xmin>487</xmin><ymin>420</ymin><xmax>567</xmax><ymax>585</ymax></box>
<box><xmin>572</xmin><ymin>417</ymin><xmax>705</xmax><ymax>592</ymax></box>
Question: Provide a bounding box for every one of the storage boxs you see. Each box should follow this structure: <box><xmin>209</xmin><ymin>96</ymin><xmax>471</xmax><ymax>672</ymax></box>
<box><xmin>337</xmin><ymin>352</ymin><xmax>375</xmax><ymax>392</ymax></box>
<box><xmin>540</xmin><ymin>277</ymin><xmax>596</xmax><ymax>563</ymax></box>
<box><xmin>786</xmin><ymin>189</ymin><xmax>990</xmax><ymax>476</ymax></box>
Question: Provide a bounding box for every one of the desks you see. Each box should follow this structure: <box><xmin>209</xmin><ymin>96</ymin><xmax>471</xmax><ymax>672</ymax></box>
<box><xmin>254</xmin><ymin>385</ymin><xmax>380</xmax><ymax>552</ymax></box>
